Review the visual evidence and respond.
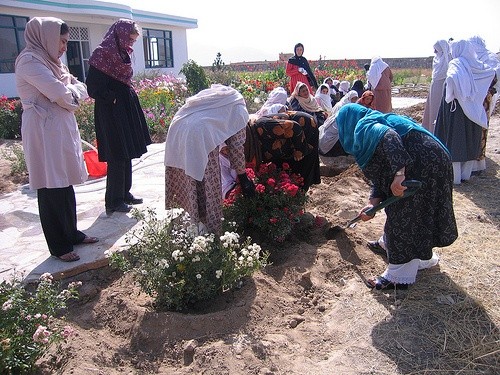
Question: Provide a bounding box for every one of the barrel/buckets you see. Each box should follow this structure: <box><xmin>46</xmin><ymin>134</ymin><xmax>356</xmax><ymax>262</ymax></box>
<box><xmin>82</xmin><ymin>150</ymin><xmax>107</xmax><ymax>176</ymax></box>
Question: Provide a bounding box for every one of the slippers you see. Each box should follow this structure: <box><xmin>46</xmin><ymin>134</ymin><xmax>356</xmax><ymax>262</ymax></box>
<box><xmin>366</xmin><ymin>239</ymin><xmax>387</xmax><ymax>256</ymax></box>
<box><xmin>53</xmin><ymin>252</ymin><xmax>80</xmax><ymax>262</ymax></box>
<box><xmin>364</xmin><ymin>275</ymin><xmax>409</xmax><ymax>291</ymax></box>
<box><xmin>79</xmin><ymin>236</ymin><xmax>99</xmax><ymax>243</ymax></box>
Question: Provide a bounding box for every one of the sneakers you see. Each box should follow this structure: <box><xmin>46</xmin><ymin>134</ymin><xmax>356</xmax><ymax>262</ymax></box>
<box><xmin>125</xmin><ymin>197</ymin><xmax>143</xmax><ymax>204</ymax></box>
<box><xmin>107</xmin><ymin>204</ymin><xmax>133</xmax><ymax>212</ymax></box>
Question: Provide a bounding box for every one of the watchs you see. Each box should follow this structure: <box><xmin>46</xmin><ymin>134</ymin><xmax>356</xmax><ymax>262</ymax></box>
<box><xmin>396</xmin><ymin>171</ymin><xmax>405</xmax><ymax>176</ymax></box>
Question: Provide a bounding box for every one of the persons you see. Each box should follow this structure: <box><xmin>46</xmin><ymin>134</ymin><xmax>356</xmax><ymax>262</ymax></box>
<box><xmin>433</xmin><ymin>40</ymin><xmax>495</xmax><ymax>185</ymax></box>
<box><xmin>422</xmin><ymin>40</ymin><xmax>452</xmax><ymax>135</ymax></box>
<box><xmin>467</xmin><ymin>36</ymin><xmax>500</xmax><ymax>172</ymax></box>
<box><xmin>87</xmin><ymin>18</ymin><xmax>152</xmax><ymax>212</ymax></box>
<box><xmin>250</xmin><ymin>111</ymin><xmax>321</xmax><ymax>194</ymax></box>
<box><xmin>367</xmin><ymin>56</ymin><xmax>393</xmax><ymax>114</ymax></box>
<box><xmin>286</xmin><ymin>43</ymin><xmax>319</xmax><ymax>97</ymax></box>
<box><xmin>336</xmin><ymin>103</ymin><xmax>458</xmax><ymax>290</ymax></box>
<box><xmin>15</xmin><ymin>16</ymin><xmax>99</xmax><ymax>261</ymax></box>
<box><xmin>164</xmin><ymin>83</ymin><xmax>249</xmax><ymax>240</ymax></box>
<box><xmin>257</xmin><ymin>77</ymin><xmax>376</xmax><ymax>157</ymax></box>
